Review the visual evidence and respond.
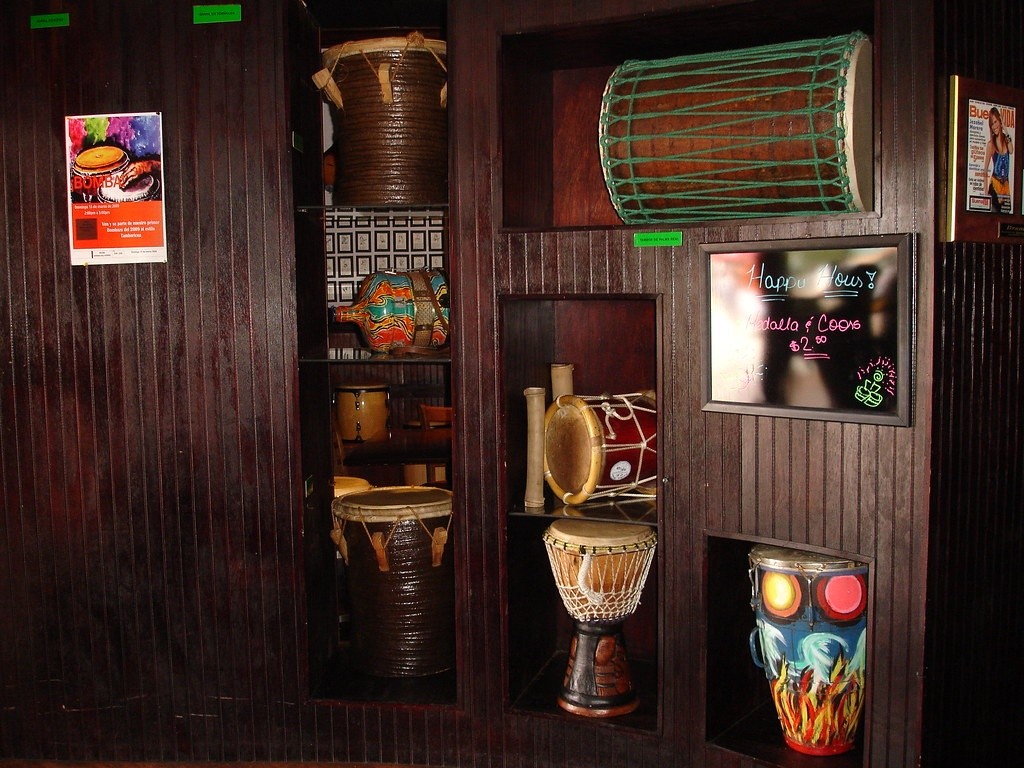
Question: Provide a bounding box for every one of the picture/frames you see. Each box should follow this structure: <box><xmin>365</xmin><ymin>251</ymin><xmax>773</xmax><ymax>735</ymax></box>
<box><xmin>695</xmin><ymin>229</ymin><xmax>921</xmax><ymax>427</ymax></box>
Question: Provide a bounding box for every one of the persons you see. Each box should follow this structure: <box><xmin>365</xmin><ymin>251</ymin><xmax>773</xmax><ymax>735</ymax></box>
<box><xmin>984</xmin><ymin>107</ymin><xmax>1013</xmax><ymax>214</ymax></box>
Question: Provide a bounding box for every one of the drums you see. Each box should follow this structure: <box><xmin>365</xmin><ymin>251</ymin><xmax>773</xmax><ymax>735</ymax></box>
<box><xmin>598</xmin><ymin>31</ymin><xmax>873</xmax><ymax>226</ymax></box>
<box><xmin>544</xmin><ymin>389</ymin><xmax>656</xmax><ymax>506</ymax></box>
<box><xmin>330</xmin><ymin>484</ymin><xmax>455</xmax><ymax>678</ymax></box>
<box><xmin>747</xmin><ymin>543</ymin><xmax>869</xmax><ymax>756</ymax></box>
<box><xmin>321</xmin><ymin>36</ymin><xmax>446</xmax><ymax>205</ymax></box>
<box><xmin>332</xmin><ymin>382</ymin><xmax>392</xmax><ymax>445</ymax></box>
<box><xmin>541</xmin><ymin>518</ymin><xmax>657</xmax><ymax>718</ymax></box>
